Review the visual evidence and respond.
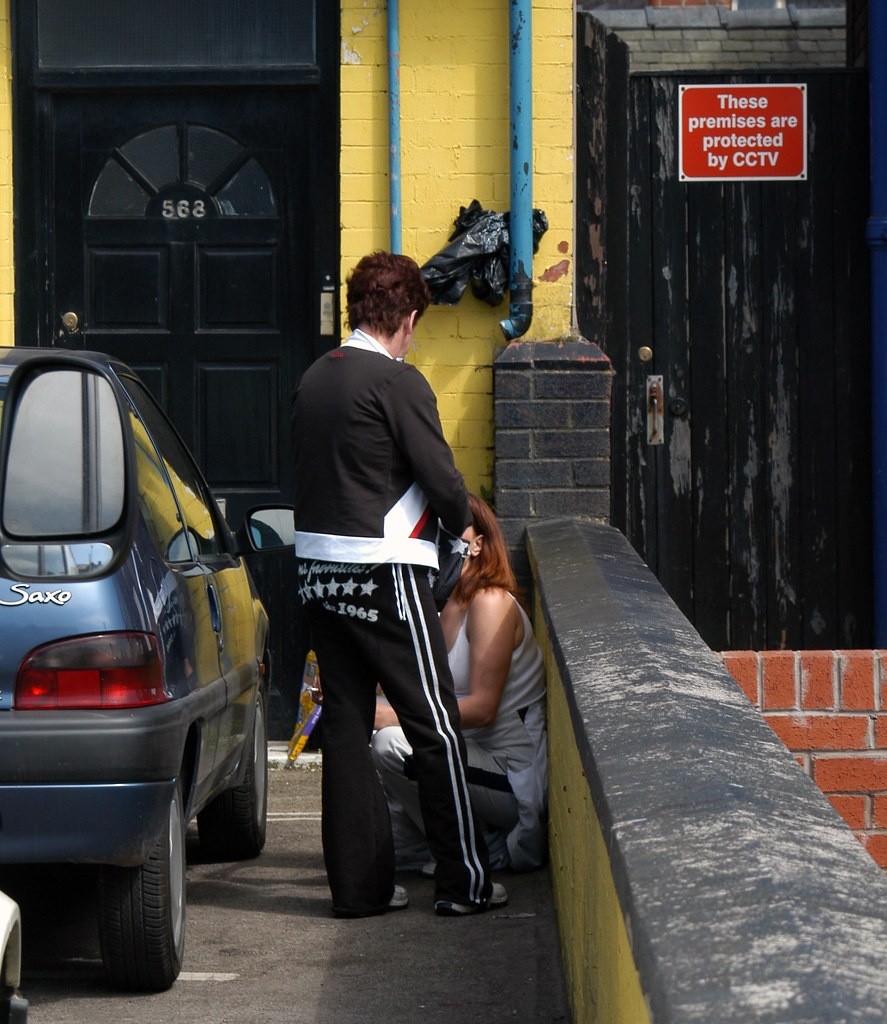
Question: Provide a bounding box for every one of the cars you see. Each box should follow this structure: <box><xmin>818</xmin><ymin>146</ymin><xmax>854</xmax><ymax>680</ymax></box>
<box><xmin>0</xmin><ymin>344</ymin><xmax>299</xmax><ymax>997</ymax></box>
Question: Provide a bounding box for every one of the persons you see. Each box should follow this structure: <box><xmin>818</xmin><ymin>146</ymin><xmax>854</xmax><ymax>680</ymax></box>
<box><xmin>293</xmin><ymin>250</ymin><xmax>506</xmax><ymax>917</ymax></box>
<box><xmin>374</xmin><ymin>494</ymin><xmax>547</xmax><ymax>874</ymax></box>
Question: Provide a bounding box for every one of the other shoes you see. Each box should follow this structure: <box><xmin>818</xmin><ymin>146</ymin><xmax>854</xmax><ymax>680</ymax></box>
<box><xmin>387</xmin><ymin>885</ymin><xmax>409</xmax><ymax>909</ymax></box>
<box><xmin>434</xmin><ymin>881</ymin><xmax>509</xmax><ymax>917</ymax></box>
<box><xmin>423</xmin><ymin>826</ymin><xmax>510</xmax><ymax>875</ymax></box>
